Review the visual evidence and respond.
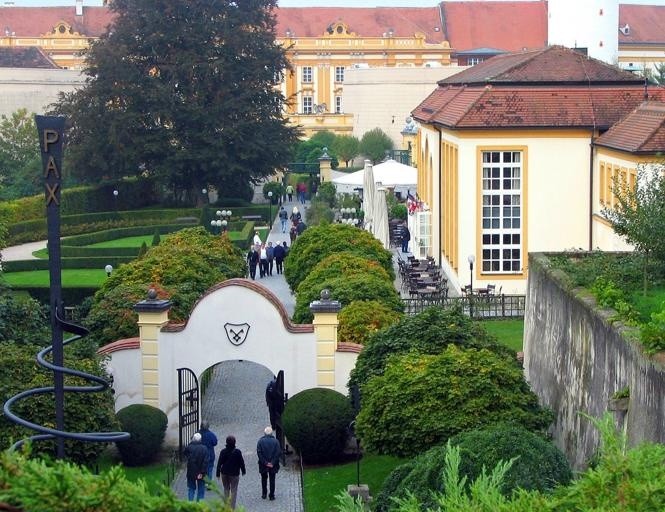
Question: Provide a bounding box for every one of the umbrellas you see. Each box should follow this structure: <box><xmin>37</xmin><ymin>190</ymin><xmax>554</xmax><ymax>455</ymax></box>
<box><xmin>334</xmin><ymin>159</ymin><xmax>418</xmax><ymax>186</ymax></box>
<box><xmin>363</xmin><ymin>161</ymin><xmax>374</xmax><ymax>231</ymax></box>
<box><xmin>373</xmin><ymin>187</ymin><xmax>391</xmax><ymax>251</ymax></box>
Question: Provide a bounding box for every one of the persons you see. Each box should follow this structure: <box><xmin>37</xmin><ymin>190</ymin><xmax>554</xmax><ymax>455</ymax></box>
<box><xmin>192</xmin><ymin>421</ymin><xmax>218</xmax><ymax>489</ymax></box>
<box><xmin>266</xmin><ymin>376</ymin><xmax>282</xmax><ymax>430</ymax></box>
<box><xmin>215</xmin><ymin>436</ymin><xmax>245</xmax><ymax>511</ymax></box>
<box><xmin>247</xmin><ymin>168</ymin><xmax>317</xmax><ymax>280</ymax></box>
<box><xmin>185</xmin><ymin>432</ymin><xmax>210</xmax><ymax>501</ymax></box>
<box><xmin>400</xmin><ymin>194</ymin><xmax>420</xmax><ymax>254</ymax></box>
<box><xmin>257</xmin><ymin>427</ymin><xmax>281</xmax><ymax>499</ymax></box>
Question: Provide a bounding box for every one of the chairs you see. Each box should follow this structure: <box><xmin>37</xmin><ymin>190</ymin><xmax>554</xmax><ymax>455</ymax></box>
<box><xmin>387</xmin><ymin>216</ymin><xmax>408</xmax><ymax>248</ymax></box>
<box><xmin>395</xmin><ymin>253</ymin><xmax>505</xmax><ymax>307</ymax></box>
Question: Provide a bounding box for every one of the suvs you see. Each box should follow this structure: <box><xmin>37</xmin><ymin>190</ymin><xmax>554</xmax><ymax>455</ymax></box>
<box><xmin>391</xmin><ymin>224</ymin><xmax>405</xmax><ymax>239</ymax></box>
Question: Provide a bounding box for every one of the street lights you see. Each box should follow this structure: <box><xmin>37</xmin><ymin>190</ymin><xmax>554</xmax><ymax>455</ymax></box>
<box><xmin>467</xmin><ymin>253</ymin><xmax>476</xmax><ymax>295</ymax></box>
<box><xmin>267</xmin><ymin>190</ymin><xmax>273</xmax><ymax>232</ymax></box>
<box><xmin>112</xmin><ymin>189</ymin><xmax>121</xmax><ymax>211</ymax></box>
<box><xmin>337</xmin><ymin>217</ymin><xmax>360</xmax><ymax>228</ymax></box>
<box><xmin>215</xmin><ymin>209</ymin><xmax>232</xmax><ymax>221</ymax></box>
<box><xmin>209</xmin><ymin>219</ymin><xmax>229</xmax><ymax>237</ymax></box>
<box><xmin>104</xmin><ymin>264</ymin><xmax>113</xmax><ymax>278</ymax></box>
<box><xmin>340</xmin><ymin>208</ymin><xmax>357</xmax><ymax>218</ymax></box>
